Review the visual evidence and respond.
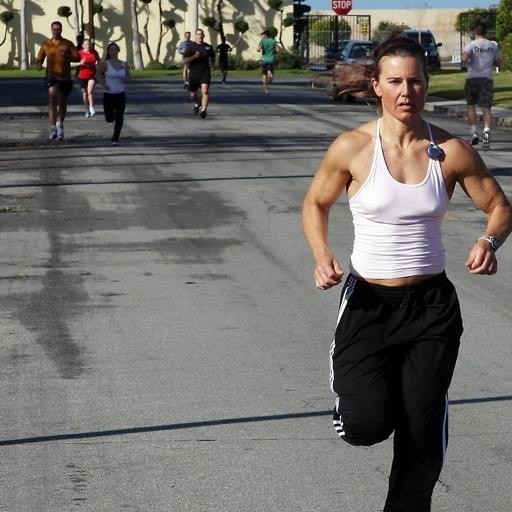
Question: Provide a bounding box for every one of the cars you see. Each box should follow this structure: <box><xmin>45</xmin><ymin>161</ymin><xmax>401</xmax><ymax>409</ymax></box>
<box><xmin>306</xmin><ymin>64</ymin><xmax>330</xmax><ymax>72</ymax></box>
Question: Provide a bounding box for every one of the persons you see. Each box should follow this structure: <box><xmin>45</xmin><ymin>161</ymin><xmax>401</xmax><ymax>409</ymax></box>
<box><xmin>183</xmin><ymin>29</ymin><xmax>216</xmax><ymax>118</ymax></box>
<box><xmin>462</xmin><ymin>24</ymin><xmax>501</xmax><ymax>148</ymax></box>
<box><xmin>216</xmin><ymin>37</ymin><xmax>232</xmax><ymax>82</ymax></box>
<box><xmin>301</xmin><ymin>37</ymin><xmax>512</xmax><ymax>512</ymax></box>
<box><xmin>257</xmin><ymin>31</ymin><xmax>279</xmax><ymax>96</ymax></box>
<box><xmin>70</xmin><ymin>39</ymin><xmax>100</xmax><ymax>118</ymax></box>
<box><xmin>178</xmin><ymin>32</ymin><xmax>194</xmax><ymax>88</ymax></box>
<box><xmin>36</xmin><ymin>22</ymin><xmax>80</xmax><ymax>140</ymax></box>
<box><xmin>96</xmin><ymin>43</ymin><xmax>128</xmax><ymax>145</ymax></box>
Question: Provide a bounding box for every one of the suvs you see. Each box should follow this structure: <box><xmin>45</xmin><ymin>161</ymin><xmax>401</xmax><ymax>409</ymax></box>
<box><xmin>326</xmin><ymin>40</ymin><xmax>382</xmax><ymax>71</ymax></box>
<box><xmin>402</xmin><ymin>28</ymin><xmax>442</xmax><ymax>72</ymax></box>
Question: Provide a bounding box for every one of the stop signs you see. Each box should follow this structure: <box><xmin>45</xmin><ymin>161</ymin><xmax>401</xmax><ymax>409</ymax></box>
<box><xmin>330</xmin><ymin>0</ymin><xmax>356</xmax><ymax>17</ymax></box>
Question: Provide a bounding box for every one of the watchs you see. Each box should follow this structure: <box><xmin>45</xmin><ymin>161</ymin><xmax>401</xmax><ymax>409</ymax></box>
<box><xmin>479</xmin><ymin>235</ymin><xmax>500</xmax><ymax>251</ymax></box>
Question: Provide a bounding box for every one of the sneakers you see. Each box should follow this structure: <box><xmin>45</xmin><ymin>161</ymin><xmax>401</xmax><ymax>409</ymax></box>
<box><xmin>472</xmin><ymin>133</ymin><xmax>480</xmax><ymax>146</ymax></box>
<box><xmin>56</xmin><ymin>121</ymin><xmax>64</xmax><ymax>142</ymax></box>
<box><xmin>48</xmin><ymin>125</ymin><xmax>57</xmax><ymax>140</ymax></box>
<box><xmin>112</xmin><ymin>139</ymin><xmax>121</xmax><ymax>146</ymax></box>
<box><xmin>193</xmin><ymin>101</ymin><xmax>202</xmax><ymax>116</ymax></box>
<box><xmin>199</xmin><ymin>108</ymin><xmax>208</xmax><ymax>119</ymax></box>
<box><xmin>480</xmin><ymin>129</ymin><xmax>491</xmax><ymax>152</ymax></box>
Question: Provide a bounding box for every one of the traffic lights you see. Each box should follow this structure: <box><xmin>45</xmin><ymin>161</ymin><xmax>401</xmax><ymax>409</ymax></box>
<box><xmin>292</xmin><ymin>18</ymin><xmax>307</xmax><ymax>31</ymax></box>
<box><xmin>293</xmin><ymin>2</ymin><xmax>311</xmax><ymax>18</ymax></box>
